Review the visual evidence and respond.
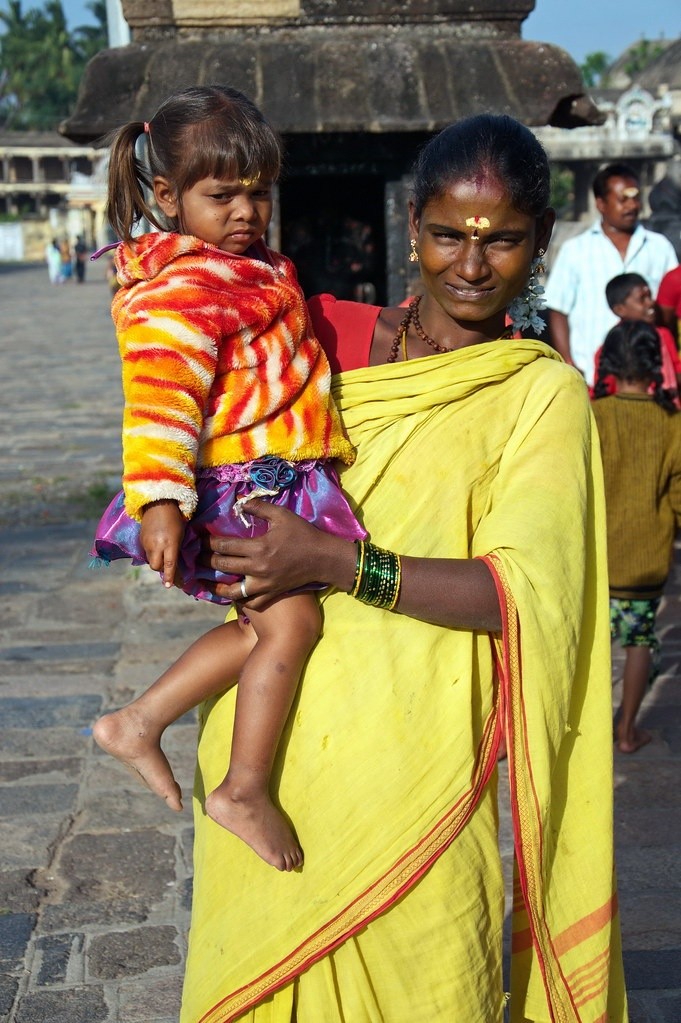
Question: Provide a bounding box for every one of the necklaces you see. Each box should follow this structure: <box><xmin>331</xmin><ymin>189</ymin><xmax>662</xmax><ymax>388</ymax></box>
<box><xmin>387</xmin><ymin>294</ymin><xmax>514</xmax><ymax>365</ymax></box>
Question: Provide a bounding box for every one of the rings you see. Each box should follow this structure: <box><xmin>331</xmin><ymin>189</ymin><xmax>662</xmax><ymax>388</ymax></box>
<box><xmin>242</xmin><ymin>581</ymin><xmax>248</xmax><ymax>597</ymax></box>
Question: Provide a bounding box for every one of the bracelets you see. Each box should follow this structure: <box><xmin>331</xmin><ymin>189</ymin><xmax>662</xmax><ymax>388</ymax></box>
<box><xmin>347</xmin><ymin>538</ymin><xmax>401</xmax><ymax>611</ymax></box>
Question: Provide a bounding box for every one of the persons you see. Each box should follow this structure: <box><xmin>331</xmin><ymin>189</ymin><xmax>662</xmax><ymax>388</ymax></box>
<box><xmin>342</xmin><ymin>163</ymin><xmax>681</xmax><ymax>407</ymax></box>
<box><xmin>47</xmin><ymin>234</ymin><xmax>87</xmax><ymax>284</ymax></box>
<box><xmin>87</xmin><ymin>84</ymin><xmax>369</xmax><ymax>871</ymax></box>
<box><xmin>591</xmin><ymin>318</ymin><xmax>681</xmax><ymax>751</ymax></box>
<box><xmin>179</xmin><ymin>114</ymin><xmax>632</xmax><ymax>1023</ymax></box>
